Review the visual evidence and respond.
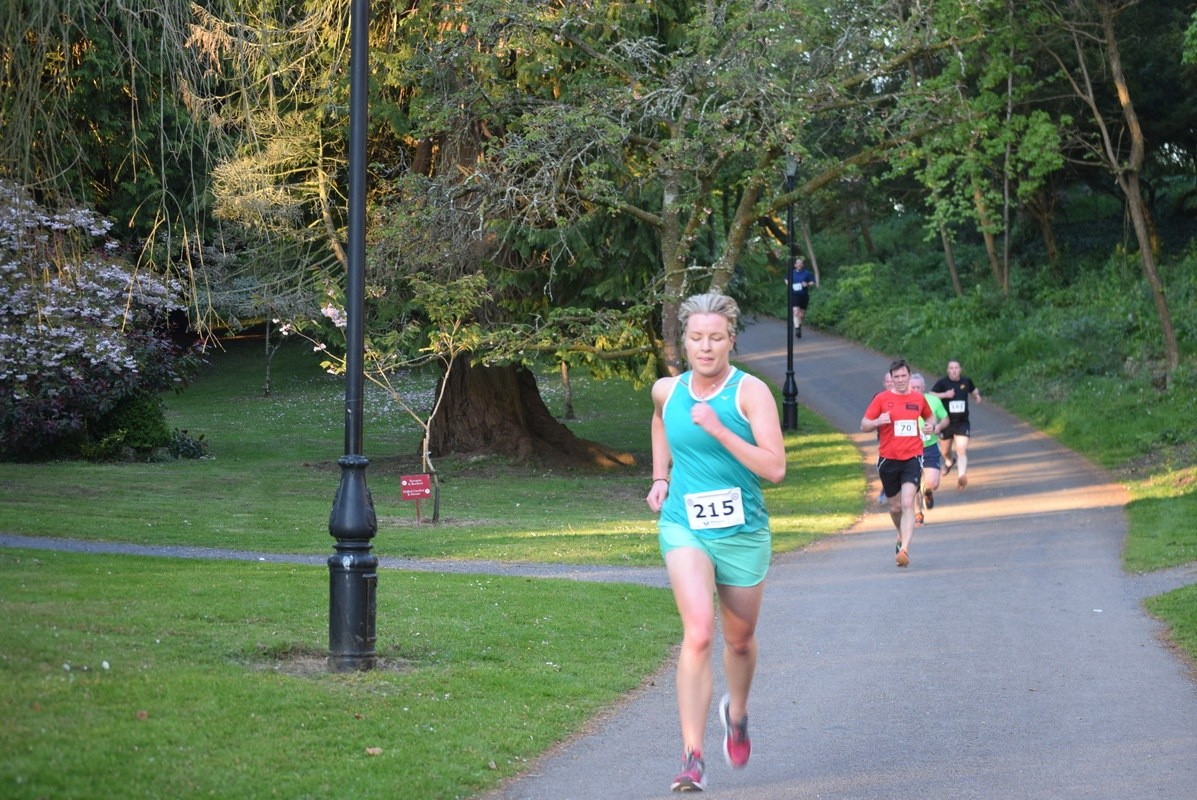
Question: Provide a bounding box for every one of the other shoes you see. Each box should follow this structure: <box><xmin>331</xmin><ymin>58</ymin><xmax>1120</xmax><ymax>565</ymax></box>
<box><xmin>795</xmin><ymin>328</ymin><xmax>801</xmax><ymax>338</ymax></box>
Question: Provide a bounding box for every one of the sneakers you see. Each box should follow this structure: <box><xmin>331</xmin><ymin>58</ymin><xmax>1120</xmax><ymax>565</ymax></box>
<box><xmin>719</xmin><ymin>695</ymin><xmax>750</xmax><ymax>768</ymax></box>
<box><xmin>897</xmin><ymin>550</ymin><xmax>909</xmax><ymax>567</ymax></box>
<box><xmin>878</xmin><ymin>490</ymin><xmax>889</xmax><ymax>505</ymax></box>
<box><xmin>671</xmin><ymin>750</ymin><xmax>707</xmax><ymax>791</ymax></box>
<box><xmin>941</xmin><ymin>457</ymin><xmax>955</xmax><ymax>477</ymax></box>
<box><xmin>956</xmin><ymin>476</ymin><xmax>967</xmax><ymax>492</ymax></box>
<box><xmin>914</xmin><ymin>513</ymin><xmax>923</xmax><ymax>526</ymax></box>
<box><xmin>923</xmin><ymin>487</ymin><xmax>933</xmax><ymax>509</ymax></box>
<box><xmin>896</xmin><ymin>539</ymin><xmax>903</xmax><ymax>553</ymax></box>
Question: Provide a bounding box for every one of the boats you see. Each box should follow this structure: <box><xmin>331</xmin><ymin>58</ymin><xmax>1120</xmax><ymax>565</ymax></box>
<box><xmin>184</xmin><ymin>252</ymin><xmax>348</xmax><ymax>347</ymax></box>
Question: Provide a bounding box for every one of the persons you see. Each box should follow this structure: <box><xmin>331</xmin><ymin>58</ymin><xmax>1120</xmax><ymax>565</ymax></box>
<box><xmin>784</xmin><ymin>259</ymin><xmax>816</xmax><ymax>339</ymax></box>
<box><xmin>645</xmin><ymin>293</ymin><xmax>786</xmax><ymax>792</ymax></box>
<box><xmin>859</xmin><ymin>359</ymin><xmax>982</xmax><ymax>569</ymax></box>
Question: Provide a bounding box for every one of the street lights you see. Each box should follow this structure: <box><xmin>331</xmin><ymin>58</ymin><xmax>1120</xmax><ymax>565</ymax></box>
<box><xmin>781</xmin><ymin>147</ymin><xmax>799</xmax><ymax>431</ymax></box>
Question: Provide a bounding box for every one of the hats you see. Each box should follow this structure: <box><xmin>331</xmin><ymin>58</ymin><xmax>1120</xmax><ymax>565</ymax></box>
<box><xmin>796</xmin><ymin>256</ymin><xmax>807</xmax><ymax>263</ymax></box>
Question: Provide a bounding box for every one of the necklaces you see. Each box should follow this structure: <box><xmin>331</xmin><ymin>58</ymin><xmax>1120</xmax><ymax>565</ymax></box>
<box><xmin>695</xmin><ymin>368</ymin><xmax>731</xmax><ymax>400</ymax></box>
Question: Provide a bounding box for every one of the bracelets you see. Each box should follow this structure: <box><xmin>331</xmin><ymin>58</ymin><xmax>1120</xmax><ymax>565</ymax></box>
<box><xmin>652</xmin><ymin>478</ymin><xmax>669</xmax><ymax>486</ymax></box>
